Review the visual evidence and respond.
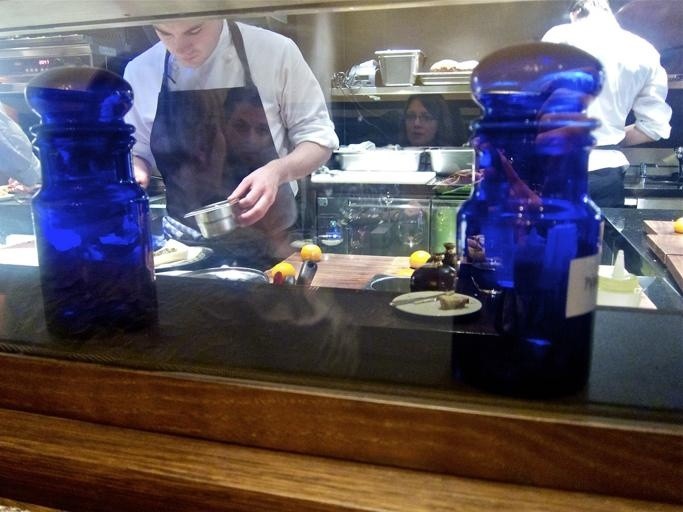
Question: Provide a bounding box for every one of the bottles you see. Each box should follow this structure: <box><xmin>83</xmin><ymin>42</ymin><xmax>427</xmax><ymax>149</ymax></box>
<box><xmin>409</xmin><ymin>242</ymin><xmax>457</xmax><ymax>292</ymax></box>
<box><xmin>22</xmin><ymin>64</ymin><xmax>162</xmax><ymax>342</ymax></box>
<box><xmin>443</xmin><ymin>40</ymin><xmax>601</xmax><ymax>408</ymax></box>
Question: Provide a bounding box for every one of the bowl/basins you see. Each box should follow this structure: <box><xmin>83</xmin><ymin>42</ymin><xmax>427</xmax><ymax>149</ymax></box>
<box><xmin>182</xmin><ymin>196</ymin><xmax>245</xmax><ymax>241</ymax></box>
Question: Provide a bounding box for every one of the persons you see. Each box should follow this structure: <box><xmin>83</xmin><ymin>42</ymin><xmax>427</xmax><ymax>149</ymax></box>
<box><xmin>0</xmin><ymin>102</ymin><xmax>42</xmax><ymax>188</ymax></box>
<box><xmin>546</xmin><ymin>0</ymin><xmax>673</xmax><ymax>209</ymax></box>
<box><xmin>401</xmin><ymin>94</ymin><xmax>468</xmax><ymax>147</ymax></box>
<box><xmin>164</xmin><ymin>86</ymin><xmax>267</xmax><ymax>236</ymax></box>
<box><xmin>123</xmin><ymin>14</ymin><xmax>339</xmax><ymax>265</ymax></box>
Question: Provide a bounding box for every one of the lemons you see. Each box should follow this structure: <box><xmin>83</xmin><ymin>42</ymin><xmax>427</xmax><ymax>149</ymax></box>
<box><xmin>271</xmin><ymin>261</ymin><xmax>296</xmax><ymax>280</ymax></box>
<box><xmin>674</xmin><ymin>218</ymin><xmax>683</xmax><ymax>233</ymax></box>
<box><xmin>409</xmin><ymin>250</ymin><xmax>431</xmax><ymax>269</ymax></box>
<box><xmin>301</xmin><ymin>244</ymin><xmax>321</xmax><ymax>262</ymax></box>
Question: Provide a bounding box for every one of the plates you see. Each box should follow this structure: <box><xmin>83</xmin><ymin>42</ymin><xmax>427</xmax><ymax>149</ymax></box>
<box><xmin>392</xmin><ymin>290</ymin><xmax>483</xmax><ymax>318</ymax></box>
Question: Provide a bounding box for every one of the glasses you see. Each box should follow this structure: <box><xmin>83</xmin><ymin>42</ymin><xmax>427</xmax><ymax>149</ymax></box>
<box><xmin>406</xmin><ymin>113</ymin><xmax>439</xmax><ymax>124</ymax></box>
